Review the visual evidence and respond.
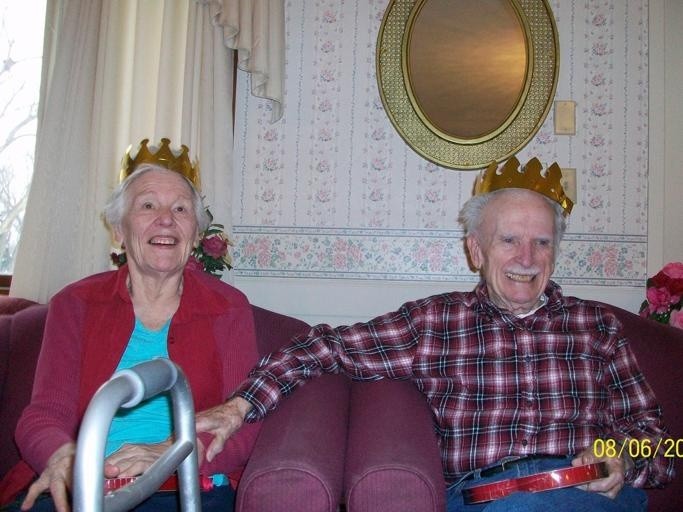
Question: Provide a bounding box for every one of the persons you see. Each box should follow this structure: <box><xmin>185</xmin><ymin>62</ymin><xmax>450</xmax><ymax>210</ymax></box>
<box><xmin>3</xmin><ymin>145</ymin><xmax>267</xmax><ymax>512</ymax></box>
<box><xmin>179</xmin><ymin>187</ymin><xmax>678</xmax><ymax>512</ymax></box>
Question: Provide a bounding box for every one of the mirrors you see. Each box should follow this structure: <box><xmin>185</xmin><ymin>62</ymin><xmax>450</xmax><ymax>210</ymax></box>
<box><xmin>375</xmin><ymin>0</ymin><xmax>560</xmax><ymax>169</ymax></box>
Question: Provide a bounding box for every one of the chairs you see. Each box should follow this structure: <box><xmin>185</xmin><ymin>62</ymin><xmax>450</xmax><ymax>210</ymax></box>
<box><xmin>346</xmin><ymin>301</ymin><xmax>683</xmax><ymax>512</ymax></box>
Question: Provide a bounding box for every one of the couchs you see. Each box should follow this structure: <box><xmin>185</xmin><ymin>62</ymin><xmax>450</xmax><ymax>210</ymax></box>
<box><xmin>0</xmin><ymin>295</ymin><xmax>353</xmax><ymax>512</ymax></box>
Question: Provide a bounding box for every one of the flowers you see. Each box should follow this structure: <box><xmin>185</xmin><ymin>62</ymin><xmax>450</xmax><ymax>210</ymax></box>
<box><xmin>108</xmin><ymin>194</ymin><xmax>235</xmax><ymax>279</ymax></box>
<box><xmin>639</xmin><ymin>261</ymin><xmax>683</xmax><ymax>328</ymax></box>
